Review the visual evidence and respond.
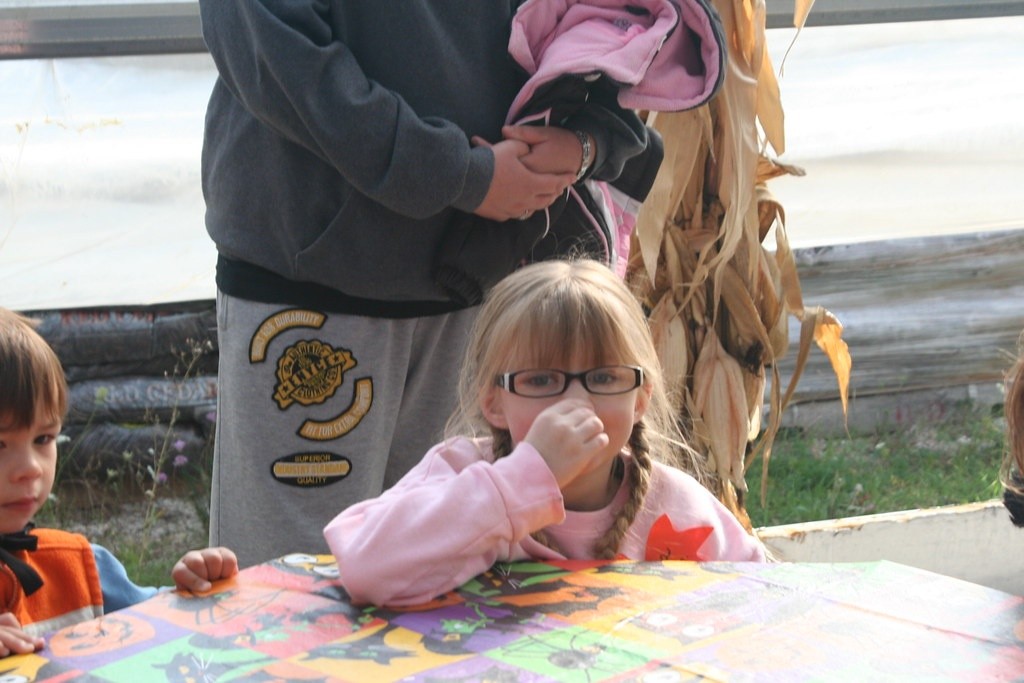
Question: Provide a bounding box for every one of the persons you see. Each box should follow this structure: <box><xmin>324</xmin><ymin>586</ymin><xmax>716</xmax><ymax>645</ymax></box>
<box><xmin>0</xmin><ymin>305</ymin><xmax>239</xmax><ymax>656</ymax></box>
<box><xmin>324</xmin><ymin>259</ymin><xmax>769</xmax><ymax>608</ymax></box>
<box><xmin>197</xmin><ymin>0</ymin><xmax>650</xmax><ymax>572</ymax></box>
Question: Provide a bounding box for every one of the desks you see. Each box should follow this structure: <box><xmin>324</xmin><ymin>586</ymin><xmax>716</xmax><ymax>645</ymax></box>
<box><xmin>0</xmin><ymin>550</ymin><xmax>1024</xmax><ymax>683</ymax></box>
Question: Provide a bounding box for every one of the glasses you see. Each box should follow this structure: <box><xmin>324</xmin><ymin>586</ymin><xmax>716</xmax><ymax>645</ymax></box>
<box><xmin>495</xmin><ymin>365</ymin><xmax>643</xmax><ymax>398</ymax></box>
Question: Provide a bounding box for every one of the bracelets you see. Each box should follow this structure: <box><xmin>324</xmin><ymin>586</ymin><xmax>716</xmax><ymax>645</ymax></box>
<box><xmin>572</xmin><ymin>131</ymin><xmax>590</xmax><ymax>178</ymax></box>
